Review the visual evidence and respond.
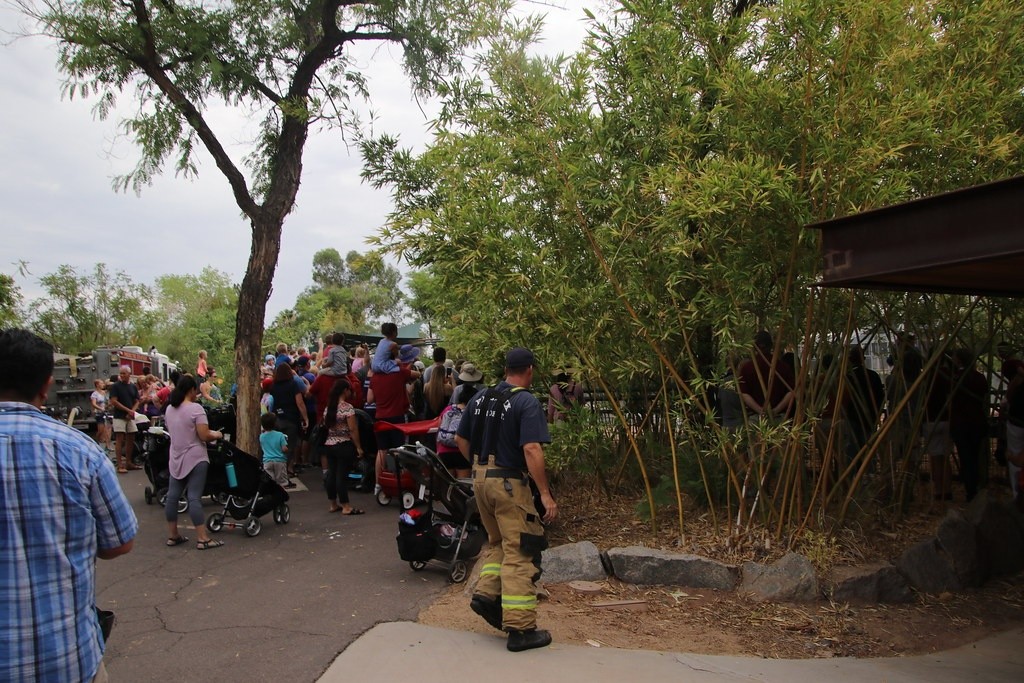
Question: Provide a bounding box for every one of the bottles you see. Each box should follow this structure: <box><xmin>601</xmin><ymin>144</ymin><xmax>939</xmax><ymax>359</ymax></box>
<box><xmin>225</xmin><ymin>462</ymin><xmax>238</xmax><ymax>487</ymax></box>
<box><xmin>416</xmin><ymin>441</ymin><xmax>428</xmax><ymax>460</ymax></box>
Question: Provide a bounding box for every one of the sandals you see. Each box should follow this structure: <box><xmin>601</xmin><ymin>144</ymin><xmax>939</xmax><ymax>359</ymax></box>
<box><xmin>166</xmin><ymin>535</ymin><xmax>187</xmax><ymax>546</ymax></box>
<box><xmin>197</xmin><ymin>538</ymin><xmax>222</xmax><ymax>550</ymax></box>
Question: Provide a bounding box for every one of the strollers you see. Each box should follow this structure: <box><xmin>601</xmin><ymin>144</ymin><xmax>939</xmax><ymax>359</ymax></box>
<box><xmin>122</xmin><ymin>410</ymin><xmax>154</xmax><ymax>466</ymax></box>
<box><xmin>371</xmin><ymin>415</ymin><xmax>441</xmax><ymax>511</ymax></box>
<box><xmin>345</xmin><ymin>407</ymin><xmax>387</xmax><ymax>493</ymax></box>
<box><xmin>205</xmin><ymin>427</ymin><xmax>291</xmax><ymax>537</ymax></box>
<box><xmin>388</xmin><ymin>440</ymin><xmax>486</xmax><ymax>584</ymax></box>
<box><xmin>137</xmin><ymin>425</ymin><xmax>229</xmax><ymax>514</ymax></box>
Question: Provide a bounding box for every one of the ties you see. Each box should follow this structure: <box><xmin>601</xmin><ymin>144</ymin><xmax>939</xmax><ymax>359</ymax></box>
<box><xmin>506</xmin><ymin>348</ymin><xmax>538</xmax><ymax>371</ymax></box>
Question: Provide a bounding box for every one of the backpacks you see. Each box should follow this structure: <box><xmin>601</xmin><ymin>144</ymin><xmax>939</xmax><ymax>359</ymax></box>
<box><xmin>557</xmin><ymin>383</ymin><xmax>577</xmax><ymax>420</ymax></box>
<box><xmin>437</xmin><ymin>406</ymin><xmax>465</xmax><ymax>447</ymax></box>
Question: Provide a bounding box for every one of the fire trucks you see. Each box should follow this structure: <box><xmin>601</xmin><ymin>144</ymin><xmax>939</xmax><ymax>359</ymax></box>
<box><xmin>40</xmin><ymin>346</ymin><xmax>183</xmax><ymax>441</ymax></box>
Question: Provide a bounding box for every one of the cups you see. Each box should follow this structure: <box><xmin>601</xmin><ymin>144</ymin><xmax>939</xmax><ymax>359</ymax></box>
<box><xmin>149</xmin><ymin>426</ymin><xmax>163</xmax><ymax>435</ymax></box>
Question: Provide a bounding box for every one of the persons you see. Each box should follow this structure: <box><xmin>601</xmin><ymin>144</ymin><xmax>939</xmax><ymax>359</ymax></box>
<box><xmin>547</xmin><ymin>364</ymin><xmax>583</xmax><ymax>424</ymax></box>
<box><xmin>196</xmin><ymin>350</ymin><xmax>209</xmax><ymax>395</ymax></box>
<box><xmin>322</xmin><ymin>379</ymin><xmax>365</xmax><ymax>515</ymax></box>
<box><xmin>163</xmin><ymin>375</ymin><xmax>224</xmax><ymax>550</ymax></box>
<box><xmin>108</xmin><ymin>365</ymin><xmax>143</xmax><ymax>473</ymax></box>
<box><xmin>136</xmin><ymin>366</ymin><xmax>181</xmax><ymax>421</ymax></box>
<box><xmin>90</xmin><ymin>378</ymin><xmax>115</xmax><ymax>452</ymax></box>
<box><xmin>258</xmin><ymin>412</ymin><xmax>289</xmax><ymax>487</ymax></box>
<box><xmin>200</xmin><ymin>366</ymin><xmax>223</xmax><ymax>406</ymax></box>
<box><xmin>436</xmin><ymin>388</ymin><xmax>480</xmax><ymax>480</ymax></box>
<box><xmin>318</xmin><ymin>322</ymin><xmax>487</xmax><ymax>496</ymax></box>
<box><xmin>0</xmin><ymin>326</ymin><xmax>138</xmax><ymax>683</ymax></box>
<box><xmin>259</xmin><ymin>335</ymin><xmax>334</xmax><ymax>474</ymax></box>
<box><xmin>454</xmin><ymin>346</ymin><xmax>557</xmax><ymax>652</ymax></box>
<box><xmin>718</xmin><ymin>332</ymin><xmax>1024</xmax><ymax>515</ymax></box>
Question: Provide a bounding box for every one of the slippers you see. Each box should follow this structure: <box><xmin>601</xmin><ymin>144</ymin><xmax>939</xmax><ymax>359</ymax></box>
<box><xmin>116</xmin><ymin>465</ymin><xmax>127</xmax><ymax>473</ymax></box>
<box><xmin>344</xmin><ymin>506</ymin><xmax>365</xmax><ymax>515</ymax></box>
<box><xmin>126</xmin><ymin>462</ymin><xmax>143</xmax><ymax>471</ymax></box>
<box><xmin>329</xmin><ymin>505</ymin><xmax>342</xmax><ymax>513</ymax></box>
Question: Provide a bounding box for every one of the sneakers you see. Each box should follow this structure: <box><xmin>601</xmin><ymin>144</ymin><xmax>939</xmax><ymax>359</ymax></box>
<box><xmin>469</xmin><ymin>597</ymin><xmax>507</xmax><ymax>630</ymax></box>
<box><xmin>506</xmin><ymin>626</ymin><xmax>552</xmax><ymax>650</ymax></box>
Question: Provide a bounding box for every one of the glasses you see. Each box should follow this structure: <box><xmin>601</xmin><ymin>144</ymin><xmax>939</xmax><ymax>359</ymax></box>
<box><xmin>211</xmin><ymin>375</ymin><xmax>217</xmax><ymax>378</ymax></box>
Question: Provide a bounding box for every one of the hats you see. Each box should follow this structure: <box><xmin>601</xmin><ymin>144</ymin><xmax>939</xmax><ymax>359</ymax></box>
<box><xmin>297</xmin><ymin>356</ymin><xmax>310</xmax><ymax>367</ymax></box>
<box><xmin>304</xmin><ymin>372</ymin><xmax>315</xmax><ymax>385</ymax></box>
<box><xmin>413</xmin><ymin>359</ymin><xmax>424</xmax><ymax>370</ymax></box>
<box><xmin>398</xmin><ymin>344</ymin><xmax>420</xmax><ymax>361</ymax></box>
<box><xmin>443</xmin><ymin>359</ymin><xmax>456</xmax><ymax>369</ymax></box>
<box><xmin>458</xmin><ymin>363</ymin><xmax>483</xmax><ymax>382</ymax></box>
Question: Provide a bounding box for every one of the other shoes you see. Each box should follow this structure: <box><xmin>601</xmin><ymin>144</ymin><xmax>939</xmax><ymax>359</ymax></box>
<box><xmin>293</xmin><ymin>462</ymin><xmax>313</xmax><ymax>470</ymax></box>
<box><xmin>285</xmin><ymin>481</ymin><xmax>297</xmax><ymax>489</ymax></box>
<box><xmin>287</xmin><ymin>471</ymin><xmax>299</xmax><ymax>477</ymax></box>
